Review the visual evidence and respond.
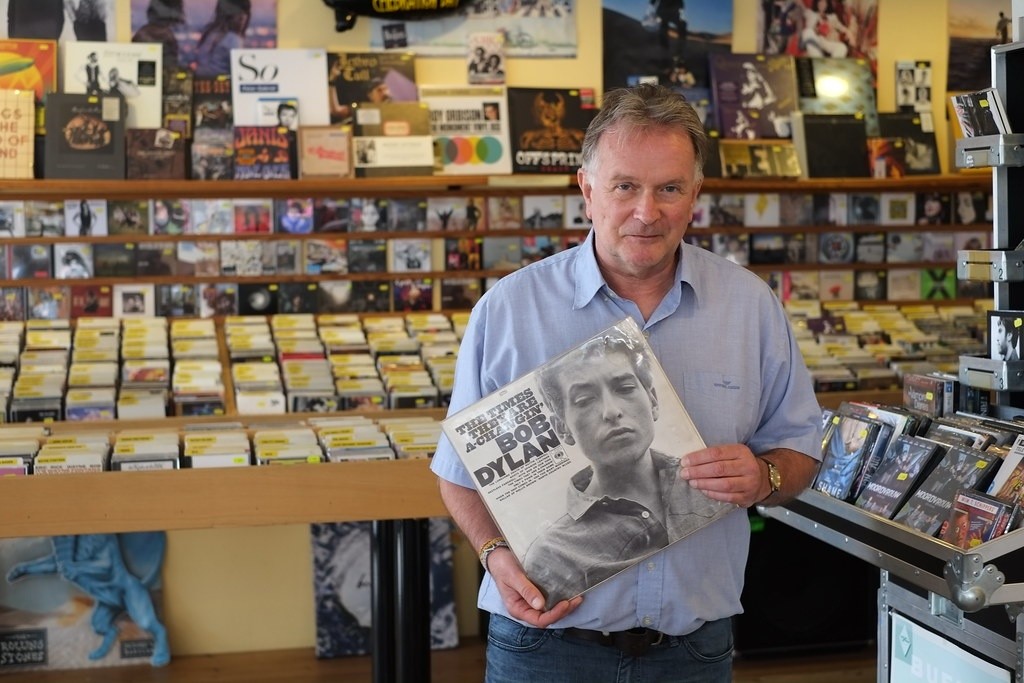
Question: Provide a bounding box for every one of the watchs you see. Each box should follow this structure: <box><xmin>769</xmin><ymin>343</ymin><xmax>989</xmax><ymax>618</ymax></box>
<box><xmin>761</xmin><ymin>458</ymin><xmax>781</xmax><ymax>502</ymax></box>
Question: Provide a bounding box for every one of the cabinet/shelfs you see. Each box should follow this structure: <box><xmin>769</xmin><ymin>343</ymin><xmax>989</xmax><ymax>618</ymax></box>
<box><xmin>0</xmin><ymin>168</ymin><xmax>992</xmax><ymax>287</ymax></box>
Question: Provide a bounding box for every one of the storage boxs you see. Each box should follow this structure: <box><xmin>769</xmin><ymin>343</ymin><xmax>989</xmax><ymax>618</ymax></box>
<box><xmin>0</xmin><ymin>610</ymin><xmax>154</xmax><ymax>672</ymax></box>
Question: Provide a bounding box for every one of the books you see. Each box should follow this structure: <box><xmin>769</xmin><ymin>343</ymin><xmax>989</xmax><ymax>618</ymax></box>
<box><xmin>439</xmin><ymin>324</ymin><xmax>739</xmax><ymax>611</ymax></box>
<box><xmin>1</xmin><ymin>0</ymin><xmax>1024</xmax><ymax>548</ymax></box>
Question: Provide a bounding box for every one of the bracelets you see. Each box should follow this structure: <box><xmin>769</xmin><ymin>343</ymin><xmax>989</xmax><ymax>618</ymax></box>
<box><xmin>479</xmin><ymin>536</ymin><xmax>508</xmax><ymax>574</ymax></box>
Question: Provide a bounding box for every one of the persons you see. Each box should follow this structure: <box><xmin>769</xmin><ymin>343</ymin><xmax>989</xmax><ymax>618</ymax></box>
<box><xmin>893</xmin><ymin>453</ymin><xmax>913</xmax><ymax>482</ymax></box>
<box><xmin>995</xmin><ymin>11</ymin><xmax>1012</xmax><ymax>44</ymax></box>
<box><xmin>651</xmin><ymin>0</ymin><xmax>695</xmax><ymax>85</ymax></box>
<box><xmin>997</xmin><ymin>317</ymin><xmax>1018</xmax><ymax>361</ymax></box>
<box><xmin>430</xmin><ymin>84</ymin><xmax>824</xmax><ymax>683</ymax></box>
<box><xmin>194</xmin><ymin>1</ymin><xmax>251</xmax><ymax>77</ymax></box>
<box><xmin>881</xmin><ymin>450</ymin><xmax>907</xmax><ymax>484</ymax></box>
<box><xmin>522</xmin><ymin>320</ymin><xmax>738</xmax><ymax>614</ymax></box>
<box><xmin>275</xmin><ymin>102</ymin><xmax>298</xmax><ymax>180</ymax></box>
<box><xmin>484</xmin><ymin>55</ymin><xmax>503</xmax><ymax>76</ymax></box>
<box><xmin>931</xmin><ymin>464</ymin><xmax>954</xmax><ymax>493</ymax></box>
<box><xmin>133</xmin><ymin>1</ymin><xmax>185</xmax><ymax>70</ymax></box>
<box><xmin>469</xmin><ymin>47</ymin><xmax>488</xmax><ymax>75</ymax></box>
<box><xmin>802</xmin><ymin>0</ymin><xmax>855</xmax><ymax>59</ymax></box>
<box><xmin>952</xmin><ymin>510</ymin><xmax>970</xmax><ymax>551</ymax></box>
<box><xmin>742</xmin><ymin>62</ymin><xmax>776</xmax><ymax>139</ymax></box>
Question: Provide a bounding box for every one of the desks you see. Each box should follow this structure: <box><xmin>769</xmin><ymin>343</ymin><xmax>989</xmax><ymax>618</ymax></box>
<box><xmin>0</xmin><ymin>407</ymin><xmax>450</xmax><ymax>683</ymax></box>
